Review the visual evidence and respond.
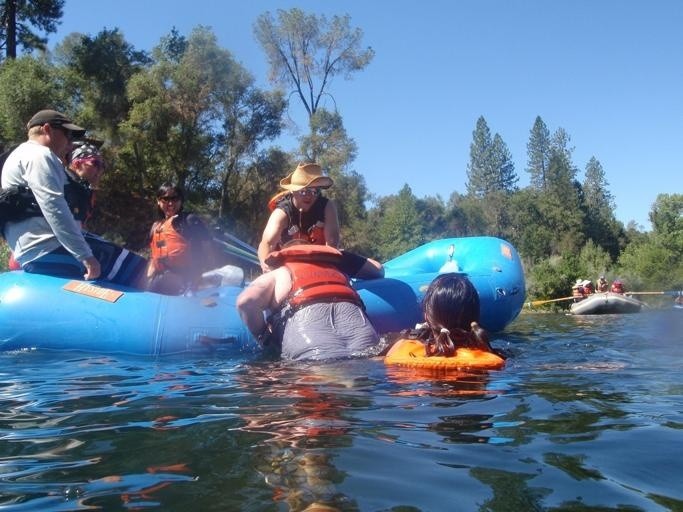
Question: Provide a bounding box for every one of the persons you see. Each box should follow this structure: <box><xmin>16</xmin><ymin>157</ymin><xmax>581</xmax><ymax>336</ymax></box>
<box><xmin>258</xmin><ymin>162</ymin><xmax>340</xmax><ymax>278</ymax></box>
<box><xmin>62</xmin><ymin>139</ymin><xmax>107</xmax><ymax>227</ymax></box>
<box><xmin>0</xmin><ymin>109</ymin><xmax>148</xmax><ymax>287</ymax></box>
<box><xmin>572</xmin><ymin>277</ymin><xmax>624</xmax><ymax>302</ymax></box>
<box><xmin>385</xmin><ymin>273</ymin><xmax>505</xmax><ymax>367</ymax></box>
<box><xmin>237</xmin><ymin>238</ymin><xmax>380</xmax><ymax>363</ymax></box>
<box><xmin>146</xmin><ymin>181</ymin><xmax>216</xmax><ymax>285</ymax></box>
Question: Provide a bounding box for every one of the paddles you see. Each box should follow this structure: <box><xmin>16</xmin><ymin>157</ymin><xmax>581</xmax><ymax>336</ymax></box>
<box><xmin>523</xmin><ymin>293</ymin><xmax>586</xmax><ymax>308</ymax></box>
<box><xmin>625</xmin><ymin>290</ymin><xmax>683</xmax><ymax>296</ymax></box>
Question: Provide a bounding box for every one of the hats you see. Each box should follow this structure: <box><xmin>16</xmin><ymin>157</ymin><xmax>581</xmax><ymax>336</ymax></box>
<box><xmin>26</xmin><ymin>109</ymin><xmax>86</xmax><ymax>142</ymax></box>
<box><xmin>280</xmin><ymin>161</ymin><xmax>334</xmax><ymax>192</ymax></box>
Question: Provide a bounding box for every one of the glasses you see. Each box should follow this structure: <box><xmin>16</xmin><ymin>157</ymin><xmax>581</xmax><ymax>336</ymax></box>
<box><xmin>297</xmin><ymin>187</ymin><xmax>322</xmax><ymax>197</ymax></box>
<box><xmin>73</xmin><ymin>157</ymin><xmax>104</xmax><ymax>170</ymax></box>
<box><xmin>158</xmin><ymin>194</ymin><xmax>180</xmax><ymax>203</ymax></box>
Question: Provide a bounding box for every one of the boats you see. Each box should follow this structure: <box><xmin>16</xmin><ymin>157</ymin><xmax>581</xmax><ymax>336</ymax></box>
<box><xmin>672</xmin><ymin>304</ymin><xmax>682</xmax><ymax>312</ymax></box>
<box><xmin>0</xmin><ymin>235</ymin><xmax>525</xmax><ymax>362</ymax></box>
<box><xmin>569</xmin><ymin>293</ymin><xmax>642</xmax><ymax>313</ymax></box>
